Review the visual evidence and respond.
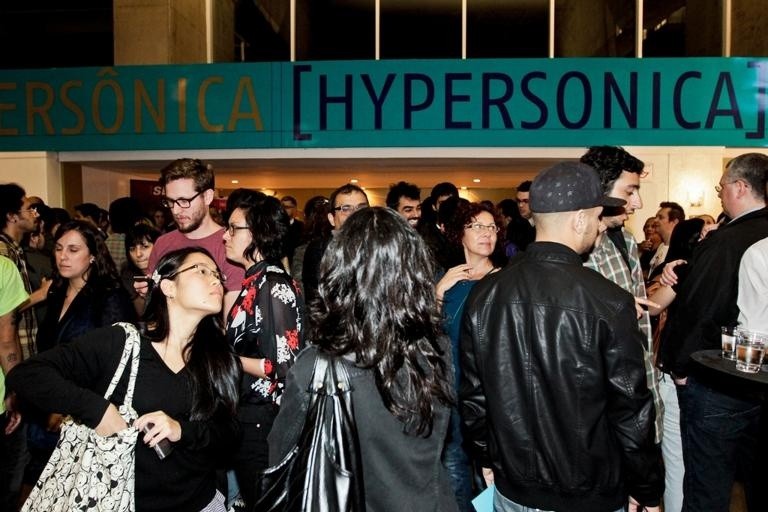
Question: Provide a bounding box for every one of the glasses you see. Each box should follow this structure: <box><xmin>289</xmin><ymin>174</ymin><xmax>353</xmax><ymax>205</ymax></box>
<box><xmin>222</xmin><ymin>222</ymin><xmax>249</xmax><ymax>236</ymax></box>
<box><xmin>464</xmin><ymin>223</ymin><xmax>500</xmax><ymax>234</ymax></box>
<box><xmin>159</xmin><ymin>192</ymin><xmax>202</xmax><ymax>208</ymax></box>
<box><xmin>332</xmin><ymin>204</ymin><xmax>369</xmax><ymax>215</ymax></box>
<box><xmin>16</xmin><ymin>207</ymin><xmax>40</xmax><ymax>218</ymax></box>
<box><xmin>714</xmin><ymin>178</ymin><xmax>747</xmax><ymax>192</ymax></box>
<box><xmin>169</xmin><ymin>263</ymin><xmax>226</xmax><ymax>282</ymax></box>
<box><xmin>284</xmin><ymin>205</ymin><xmax>295</xmax><ymax>209</ymax></box>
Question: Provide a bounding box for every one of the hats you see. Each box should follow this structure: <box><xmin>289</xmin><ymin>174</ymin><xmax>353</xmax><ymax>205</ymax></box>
<box><xmin>528</xmin><ymin>160</ymin><xmax>626</xmax><ymax>213</ymax></box>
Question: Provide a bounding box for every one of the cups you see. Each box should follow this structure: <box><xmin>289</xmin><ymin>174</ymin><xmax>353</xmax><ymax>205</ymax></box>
<box><xmin>733</xmin><ymin>331</ymin><xmax>767</xmax><ymax>375</ymax></box>
<box><xmin>719</xmin><ymin>325</ymin><xmax>749</xmax><ymax>363</ymax></box>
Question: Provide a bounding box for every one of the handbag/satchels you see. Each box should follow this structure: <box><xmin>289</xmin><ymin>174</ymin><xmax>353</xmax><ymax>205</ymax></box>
<box><xmin>20</xmin><ymin>320</ymin><xmax>141</xmax><ymax>512</ymax></box>
<box><xmin>251</xmin><ymin>347</ymin><xmax>366</xmax><ymax>512</ymax></box>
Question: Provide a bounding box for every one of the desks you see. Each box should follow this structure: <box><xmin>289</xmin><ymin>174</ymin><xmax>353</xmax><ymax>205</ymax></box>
<box><xmin>688</xmin><ymin>346</ymin><xmax>768</xmax><ymax>400</ymax></box>
